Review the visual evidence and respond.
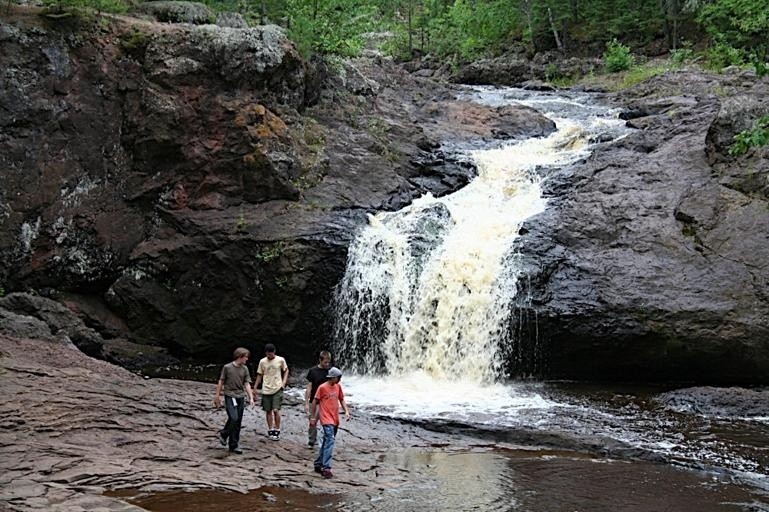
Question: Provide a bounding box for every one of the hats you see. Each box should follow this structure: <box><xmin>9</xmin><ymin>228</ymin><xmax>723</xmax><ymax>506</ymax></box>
<box><xmin>326</xmin><ymin>367</ymin><xmax>343</xmax><ymax>378</ymax></box>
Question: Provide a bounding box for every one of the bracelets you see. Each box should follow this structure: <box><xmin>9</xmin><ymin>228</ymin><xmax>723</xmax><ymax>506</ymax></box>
<box><xmin>310</xmin><ymin>418</ymin><xmax>316</xmax><ymax>420</ymax></box>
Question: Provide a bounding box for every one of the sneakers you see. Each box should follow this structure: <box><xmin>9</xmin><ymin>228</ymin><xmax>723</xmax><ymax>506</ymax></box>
<box><xmin>215</xmin><ymin>430</ymin><xmax>227</xmax><ymax>446</ymax></box>
<box><xmin>268</xmin><ymin>428</ymin><xmax>281</xmax><ymax>441</ymax></box>
<box><xmin>307</xmin><ymin>443</ymin><xmax>313</xmax><ymax>449</ymax></box>
<box><xmin>314</xmin><ymin>465</ymin><xmax>333</xmax><ymax>478</ymax></box>
<box><xmin>228</xmin><ymin>446</ymin><xmax>243</xmax><ymax>454</ymax></box>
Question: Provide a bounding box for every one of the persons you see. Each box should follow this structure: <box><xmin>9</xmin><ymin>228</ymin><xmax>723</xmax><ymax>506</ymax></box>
<box><xmin>213</xmin><ymin>347</ymin><xmax>256</xmax><ymax>455</ymax></box>
<box><xmin>310</xmin><ymin>366</ymin><xmax>351</xmax><ymax>479</ymax></box>
<box><xmin>252</xmin><ymin>343</ymin><xmax>290</xmax><ymax>442</ymax></box>
<box><xmin>303</xmin><ymin>351</ymin><xmax>332</xmax><ymax>450</ymax></box>
